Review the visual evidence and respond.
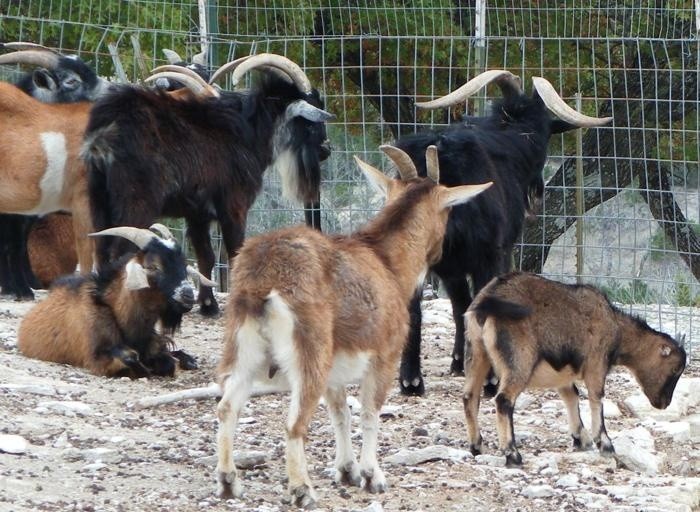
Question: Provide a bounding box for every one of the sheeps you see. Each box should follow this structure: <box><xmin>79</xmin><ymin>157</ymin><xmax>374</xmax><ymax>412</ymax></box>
<box><xmin>0</xmin><ymin>40</ymin><xmax>336</xmax><ymax>303</ymax></box>
<box><xmin>458</xmin><ymin>267</ymin><xmax>690</xmax><ymax>469</ymax></box>
<box><xmin>213</xmin><ymin>144</ymin><xmax>497</xmax><ymax>512</ymax></box>
<box><xmin>15</xmin><ymin>219</ymin><xmax>218</xmax><ymax>380</ymax></box>
<box><xmin>377</xmin><ymin>67</ymin><xmax>615</xmax><ymax>399</ymax></box>
